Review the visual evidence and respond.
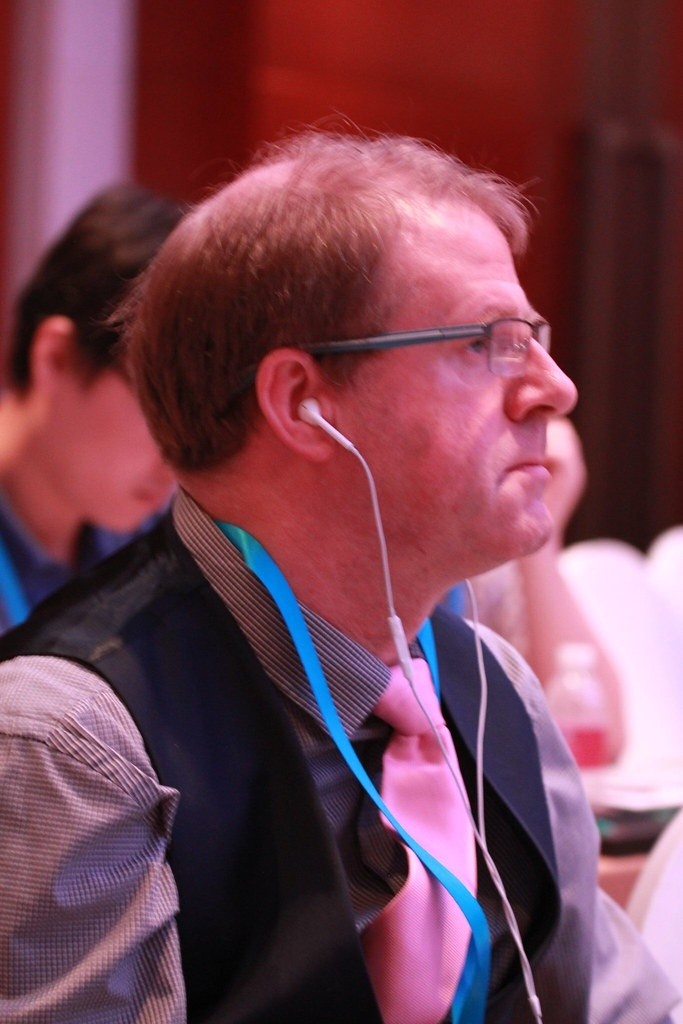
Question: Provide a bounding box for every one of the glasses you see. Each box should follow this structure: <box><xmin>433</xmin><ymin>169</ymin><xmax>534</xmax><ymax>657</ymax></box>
<box><xmin>236</xmin><ymin>316</ymin><xmax>553</xmax><ymax>379</ymax></box>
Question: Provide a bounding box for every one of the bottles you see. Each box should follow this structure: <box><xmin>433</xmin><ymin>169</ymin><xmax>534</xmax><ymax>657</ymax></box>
<box><xmin>546</xmin><ymin>641</ymin><xmax>615</xmax><ymax>773</ymax></box>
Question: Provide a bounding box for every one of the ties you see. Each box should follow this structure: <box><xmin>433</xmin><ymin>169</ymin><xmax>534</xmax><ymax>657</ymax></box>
<box><xmin>356</xmin><ymin>658</ymin><xmax>478</xmax><ymax>1024</ymax></box>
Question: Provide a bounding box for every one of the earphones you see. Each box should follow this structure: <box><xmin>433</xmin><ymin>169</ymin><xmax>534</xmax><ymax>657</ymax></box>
<box><xmin>297</xmin><ymin>397</ymin><xmax>354</xmax><ymax>451</ymax></box>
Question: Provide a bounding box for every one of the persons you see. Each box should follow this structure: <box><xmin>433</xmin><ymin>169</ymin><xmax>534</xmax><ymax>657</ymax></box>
<box><xmin>0</xmin><ymin>135</ymin><xmax>683</xmax><ymax>1024</ymax></box>
<box><xmin>0</xmin><ymin>184</ymin><xmax>624</xmax><ymax>769</ymax></box>
